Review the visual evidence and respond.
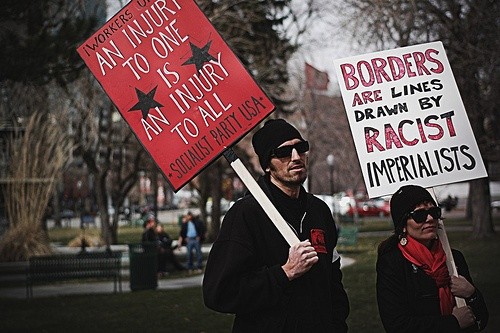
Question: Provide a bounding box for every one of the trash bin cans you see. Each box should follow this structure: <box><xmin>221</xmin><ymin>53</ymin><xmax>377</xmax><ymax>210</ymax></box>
<box><xmin>128</xmin><ymin>242</ymin><xmax>162</xmax><ymax>290</ymax></box>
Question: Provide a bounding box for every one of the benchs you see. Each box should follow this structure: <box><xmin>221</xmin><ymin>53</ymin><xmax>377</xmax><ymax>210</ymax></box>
<box><xmin>25</xmin><ymin>251</ymin><xmax>122</xmax><ymax>299</ymax></box>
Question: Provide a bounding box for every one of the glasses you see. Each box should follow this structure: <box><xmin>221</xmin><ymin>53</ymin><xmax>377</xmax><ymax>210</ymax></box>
<box><xmin>410</xmin><ymin>207</ymin><xmax>441</xmax><ymax>223</ymax></box>
<box><xmin>273</xmin><ymin>141</ymin><xmax>309</xmax><ymax>159</ymax></box>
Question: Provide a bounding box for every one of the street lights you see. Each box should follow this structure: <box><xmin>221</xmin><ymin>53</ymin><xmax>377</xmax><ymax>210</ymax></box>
<box><xmin>326</xmin><ymin>155</ymin><xmax>335</xmax><ymax>196</ymax></box>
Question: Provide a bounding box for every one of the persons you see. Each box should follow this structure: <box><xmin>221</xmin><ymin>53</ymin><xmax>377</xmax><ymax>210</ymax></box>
<box><xmin>376</xmin><ymin>185</ymin><xmax>488</xmax><ymax>333</ymax></box>
<box><xmin>141</xmin><ymin>220</ymin><xmax>184</xmax><ymax>274</ymax></box>
<box><xmin>178</xmin><ymin>210</ymin><xmax>206</xmax><ymax>273</ymax></box>
<box><xmin>203</xmin><ymin>118</ymin><xmax>350</xmax><ymax>333</ymax></box>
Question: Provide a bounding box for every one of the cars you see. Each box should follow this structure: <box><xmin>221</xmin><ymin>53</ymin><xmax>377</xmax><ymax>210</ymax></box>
<box><xmin>348</xmin><ymin>199</ymin><xmax>390</xmax><ymax>217</ymax></box>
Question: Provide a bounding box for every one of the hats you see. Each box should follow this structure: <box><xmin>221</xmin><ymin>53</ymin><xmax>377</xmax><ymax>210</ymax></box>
<box><xmin>252</xmin><ymin>119</ymin><xmax>305</xmax><ymax>172</ymax></box>
<box><xmin>391</xmin><ymin>185</ymin><xmax>437</xmax><ymax>229</ymax></box>
